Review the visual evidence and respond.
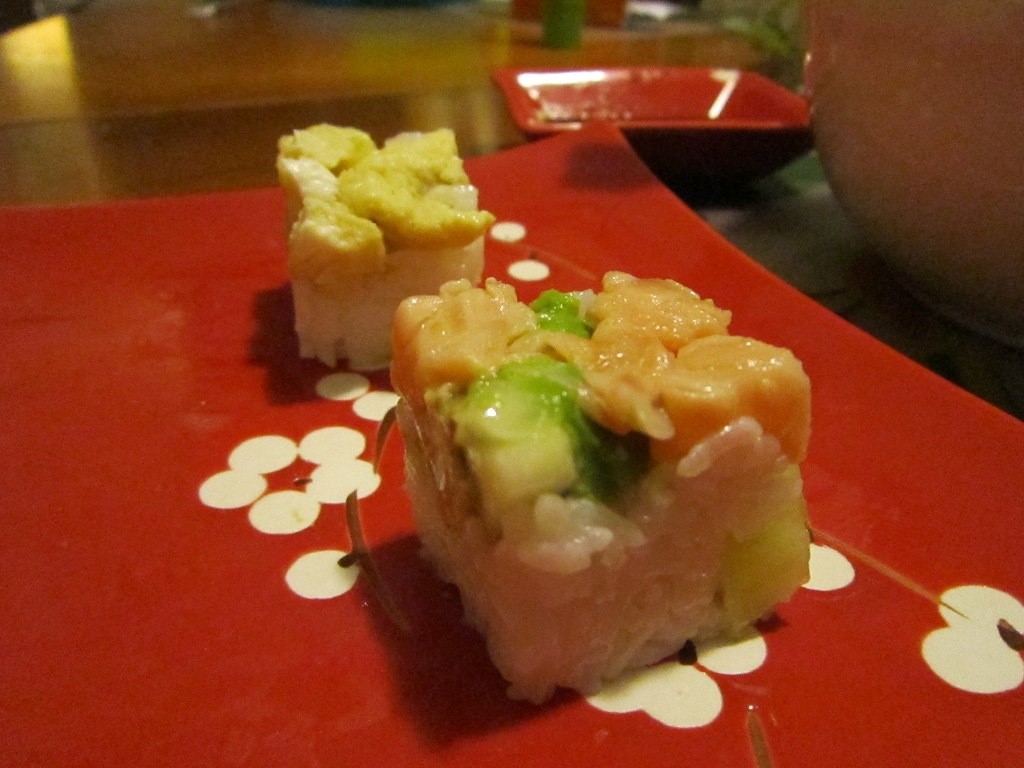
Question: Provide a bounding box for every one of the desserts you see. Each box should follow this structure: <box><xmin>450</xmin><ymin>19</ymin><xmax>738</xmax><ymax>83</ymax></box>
<box><xmin>276</xmin><ymin>124</ymin><xmax>495</xmax><ymax>370</ymax></box>
<box><xmin>389</xmin><ymin>272</ymin><xmax>813</xmax><ymax>704</ymax></box>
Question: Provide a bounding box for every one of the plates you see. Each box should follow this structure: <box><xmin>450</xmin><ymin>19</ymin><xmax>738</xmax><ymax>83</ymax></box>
<box><xmin>0</xmin><ymin>122</ymin><xmax>1022</xmax><ymax>768</ymax></box>
<box><xmin>489</xmin><ymin>61</ymin><xmax>815</xmax><ymax>198</ymax></box>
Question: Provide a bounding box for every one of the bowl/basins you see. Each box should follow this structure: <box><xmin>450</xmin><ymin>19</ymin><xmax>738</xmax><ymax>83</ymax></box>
<box><xmin>795</xmin><ymin>0</ymin><xmax>1023</xmax><ymax>348</ymax></box>
